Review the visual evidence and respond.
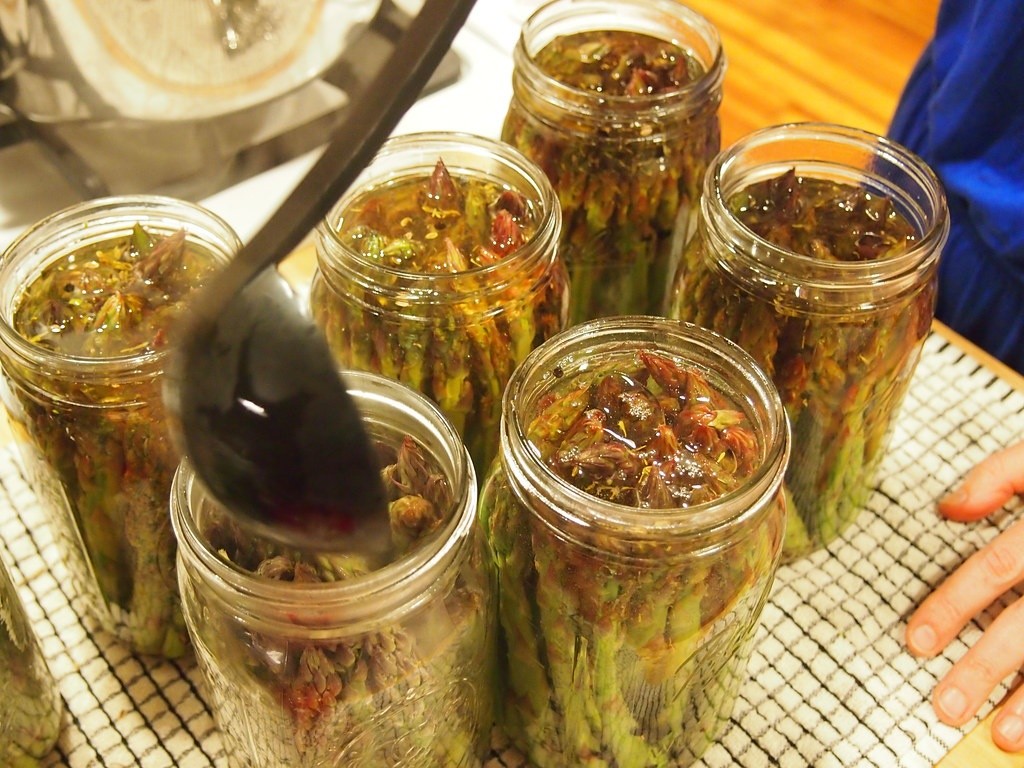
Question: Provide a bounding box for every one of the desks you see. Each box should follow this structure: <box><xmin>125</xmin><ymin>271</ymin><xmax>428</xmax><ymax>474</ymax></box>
<box><xmin>1</xmin><ymin>2</ymin><xmax>1023</xmax><ymax>766</ymax></box>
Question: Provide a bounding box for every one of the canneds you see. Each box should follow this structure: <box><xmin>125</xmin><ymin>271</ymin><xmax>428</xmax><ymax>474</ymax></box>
<box><xmin>0</xmin><ymin>0</ymin><xmax>951</xmax><ymax>768</ymax></box>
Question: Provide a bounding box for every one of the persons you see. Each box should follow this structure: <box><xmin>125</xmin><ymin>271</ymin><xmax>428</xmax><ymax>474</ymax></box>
<box><xmin>860</xmin><ymin>1</ymin><xmax>1023</xmax><ymax>753</ymax></box>
